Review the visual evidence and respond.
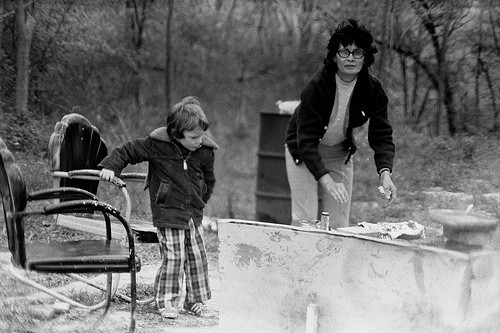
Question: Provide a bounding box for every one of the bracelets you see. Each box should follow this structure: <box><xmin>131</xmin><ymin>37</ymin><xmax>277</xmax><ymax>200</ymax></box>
<box><xmin>379</xmin><ymin>169</ymin><xmax>391</xmax><ymax>177</ymax></box>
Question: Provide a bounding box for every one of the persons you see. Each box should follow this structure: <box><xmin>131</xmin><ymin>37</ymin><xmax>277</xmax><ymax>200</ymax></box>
<box><xmin>99</xmin><ymin>96</ymin><xmax>219</xmax><ymax>319</ymax></box>
<box><xmin>284</xmin><ymin>19</ymin><xmax>397</xmax><ymax>229</ymax></box>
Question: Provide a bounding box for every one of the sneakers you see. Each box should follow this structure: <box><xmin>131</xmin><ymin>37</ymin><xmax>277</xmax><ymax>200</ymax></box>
<box><xmin>160</xmin><ymin>307</ymin><xmax>179</xmax><ymax>319</ymax></box>
<box><xmin>183</xmin><ymin>302</ymin><xmax>215</xmax><ymax>318</ymax></box>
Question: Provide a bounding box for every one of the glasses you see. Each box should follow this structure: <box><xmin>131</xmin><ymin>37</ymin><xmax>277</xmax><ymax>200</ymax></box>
<box><xmin>337</xmin><ymin>49</ymin><xmax>366</xmax><ymax>58</ymax></box>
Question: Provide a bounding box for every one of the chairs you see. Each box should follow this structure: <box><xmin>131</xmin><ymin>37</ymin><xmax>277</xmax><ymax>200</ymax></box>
<box><xmin>48</xmin><ymin>113</ymin><xmax>160</xmax><ymax>305</ymax></box>
<box><xmin>0</xmin><ymin>139</ymin><xmax>141</xmax><ymax>333</ymax></box>
